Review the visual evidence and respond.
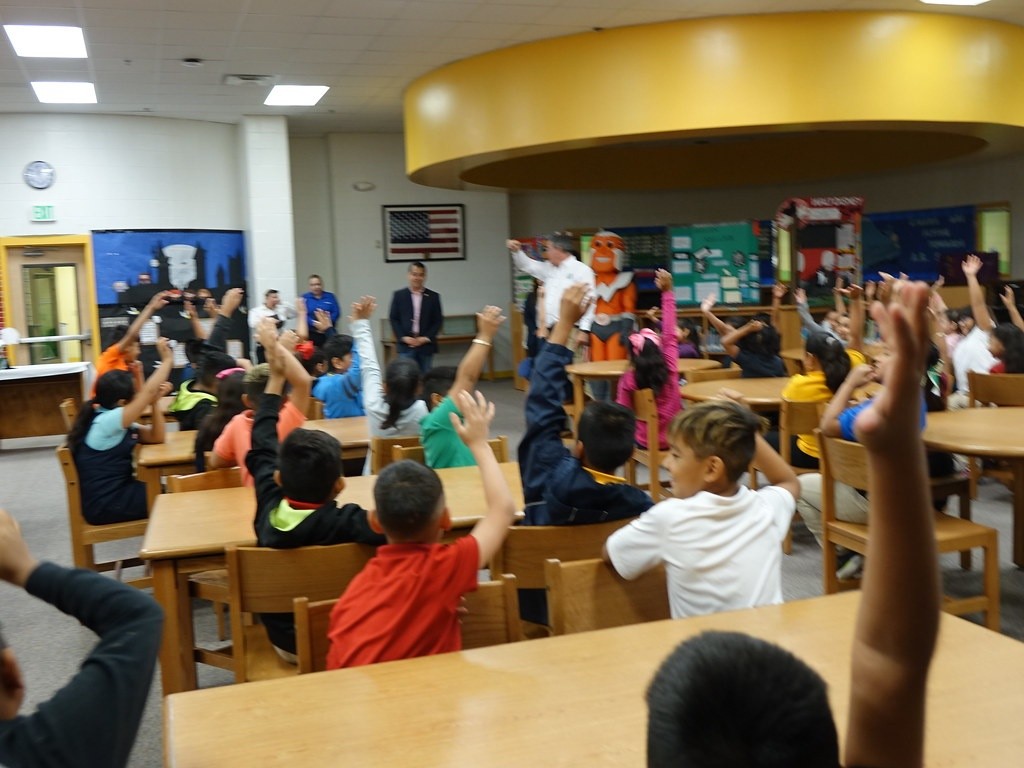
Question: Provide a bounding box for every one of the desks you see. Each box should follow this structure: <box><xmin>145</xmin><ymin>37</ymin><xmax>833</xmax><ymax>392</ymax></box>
<box><xmin>778</xmin><ymin>349</ymin><xmax>807</xmax><ymax>374</ymax></box>
<box><xmin>379</xmin><ymin>312</ymin><xmax>495</xmax><ymax>383</ymax></box>
<box><xmin>141</xmin><ymin>461</ymin><xmax>551</xmax><ymax>690</ymax></box>
<box><xmin>138</xmin><ymin>416</ymin><xmax>370</xmax><ymax>510</ymax></box>
<box><xmin>162</xmin><ymin>590</ymin><xmax>1023</xmax><ymax>767</ymax></box>
<box><xmin>565</xmin><ymin>357</ymin><xmax>717</xmax><ymax>445</ymax></box>
<box><xmin>677</xmin><ymin>376</ymin><xmax>796</xmax><ymax>487</ymax></box>
<box><xmin>918</xmin><ymin>408</ymin><xmax>1023</xmax><ymax>568</ymax></box>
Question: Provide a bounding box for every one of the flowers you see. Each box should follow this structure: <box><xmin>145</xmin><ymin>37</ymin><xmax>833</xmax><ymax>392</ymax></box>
<box><xmin>294</xmin><ymin>336</ymin><xmax>315</xmax><ymax>359</ymax></box>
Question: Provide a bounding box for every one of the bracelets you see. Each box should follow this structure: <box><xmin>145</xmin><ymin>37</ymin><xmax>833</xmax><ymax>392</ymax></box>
<box><xmin>472</xmin><ymin>337</ymin><xmax>493</xmax><ymax>347</ymax></box>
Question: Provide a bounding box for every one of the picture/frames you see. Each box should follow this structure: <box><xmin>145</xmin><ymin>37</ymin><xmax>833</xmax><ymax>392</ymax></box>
<box><xmin>383</xmin><ymin>201</ymin><xmax>464</xmax><ymax>263</ymax></box>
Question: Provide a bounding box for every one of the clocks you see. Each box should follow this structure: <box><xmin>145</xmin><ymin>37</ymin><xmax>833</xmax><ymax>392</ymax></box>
<box><xmin>24</xmin><ymin>161</ymin><xmax>55</xmax><ymax>188</ymax></box>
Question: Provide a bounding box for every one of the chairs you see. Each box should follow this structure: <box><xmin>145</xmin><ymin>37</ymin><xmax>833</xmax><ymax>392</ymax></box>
<box><xmin>50</xmin><ymin>361</ymin><xmax>1023</xmax><ymax>768</ymax></box>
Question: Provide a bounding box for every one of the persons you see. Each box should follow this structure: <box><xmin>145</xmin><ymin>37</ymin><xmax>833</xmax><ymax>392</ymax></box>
<box><xmin>0</xmin><ymin>511</ymin><xmax>164</xmax><ymax>768</ymax></box>
<box><xmin>66</xmin><ymin>234</ymin><xmax>1024</xmax><ymax>671</ymax></box>
<box><xmin>642</xmin><ymin>279</ymin><xmax>940</xmax><ymax>768</ymax></box>
